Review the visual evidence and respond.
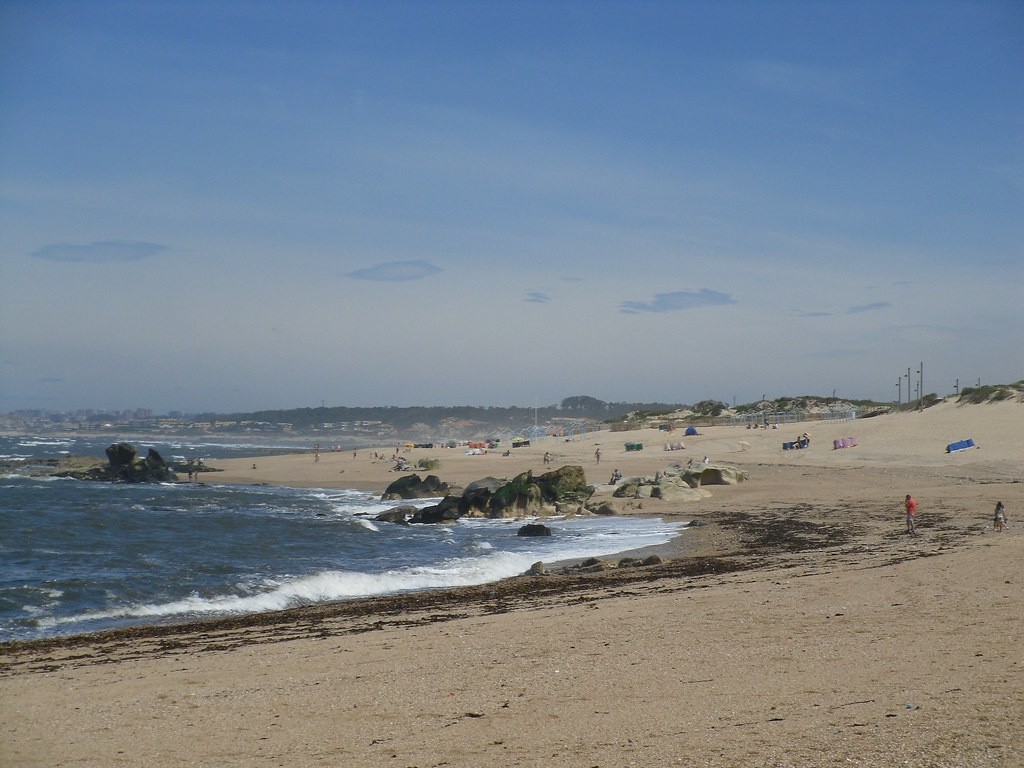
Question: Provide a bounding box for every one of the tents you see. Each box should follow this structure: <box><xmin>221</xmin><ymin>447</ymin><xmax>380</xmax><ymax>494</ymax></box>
<box><xmin>833</xmin><ymin>435</ymin><xmax>857</xmax><ymax>450</ymax></box>
<box><xmin>945</xmin><ymin>438</ymin><xmax>976</xmax><ymax>452</ymax></box>
<box><xmin>625</xmin><ymin>441</ymin><xmax>643</xmax><ymax>452</ymax></box>
<box><xmin>684</xmin><ymin>425</ymin><xmax>698</xmax><ymax>435</ymax></box>
<box><xmin>405</xmin><ymin>436</ymin><xmax>530</xmax><ymax>448</ymax></box>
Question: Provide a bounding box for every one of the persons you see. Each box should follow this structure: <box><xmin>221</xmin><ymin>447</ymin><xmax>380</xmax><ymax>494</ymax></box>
<box><xmin>785</xmin><ymin>432</ymin><xmax>810</xmax><ymax>450</ymax></box>
<box><xmin>746</xmin><ymin>417</ymin><xmax>778</xmax><ymax>430</ymax></box>
<box><xmin>594</xmin><ymin>441</ymin><xmax>710</xmax><ymax>484</ymax></box>
<box><xmin>993</xmin><ymin>501</ymin><xmax>1011</xmax><ymax>533</ymax></box>
<box><xmin>371</xmin><ymin>442</ymin><xmax>551</xmax><ymax>471</ymax></box>
<box><xmin>187</xmin><ymin>458</ymin><xmax>257</xmax><ymax>483</ymax></box>
<box><xmin>903</xmin><ymin>494</ymin><xmax>916</xmax><ymax>533</ymax></box>
<box><xmin>313</xmin><ymin>441</ymin><xmax>359</xmax><ymax>464</ymax></box>
